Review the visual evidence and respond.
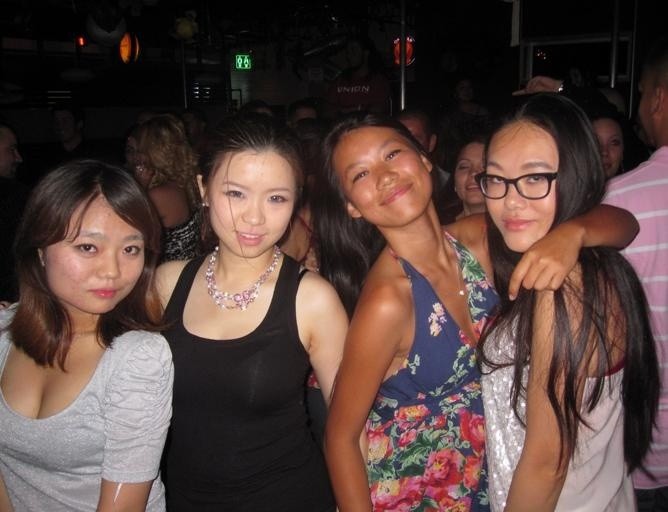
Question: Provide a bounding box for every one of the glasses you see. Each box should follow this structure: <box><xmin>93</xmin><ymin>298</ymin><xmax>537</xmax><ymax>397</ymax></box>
<box><xmin>471</xmin><ymin>168</ymin><xmax>558</xmax><ymax>201</ymax></box>
